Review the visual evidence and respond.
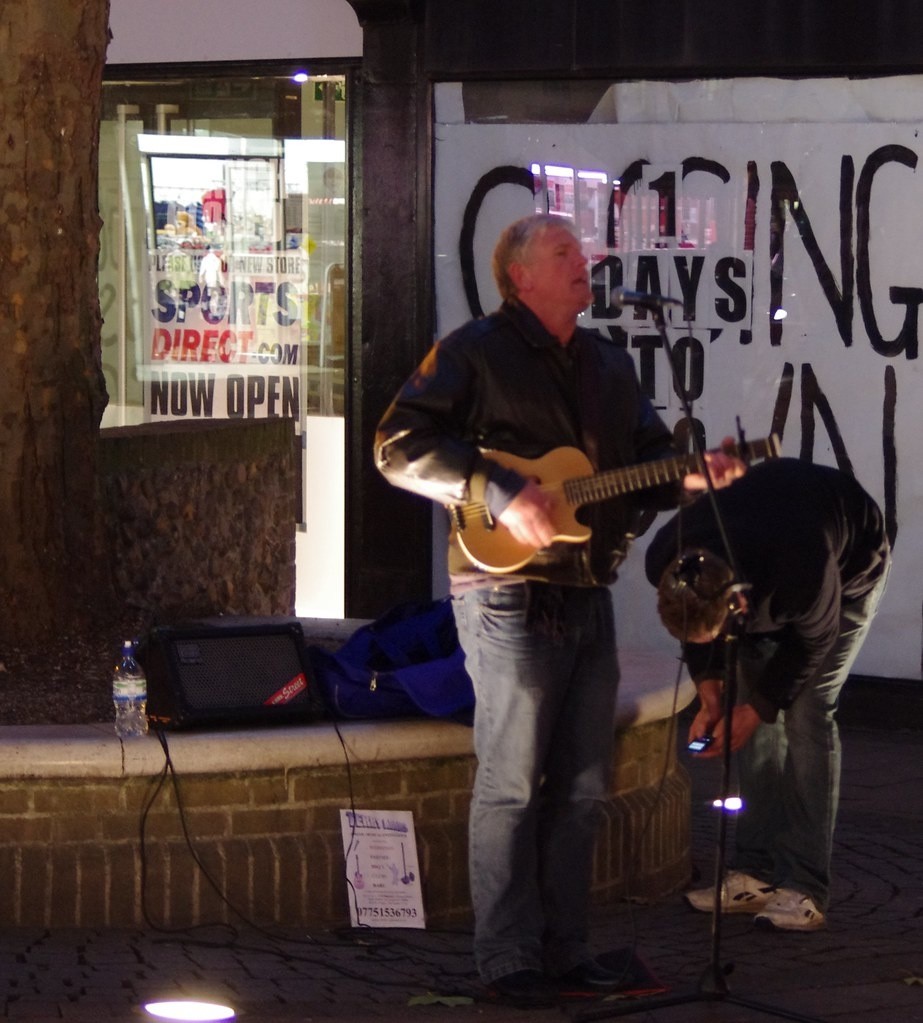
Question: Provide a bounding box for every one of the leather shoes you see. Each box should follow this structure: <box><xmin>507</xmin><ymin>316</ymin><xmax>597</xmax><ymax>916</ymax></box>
<box><xmin>479</xmin><ymin>970</ymin><xmax>552</xmax><ymax>1009</ymax></box>
<box><xmin>545</xmin><ymin>957</ymin><xmax>633</xmax><ymax>992</ymax></box>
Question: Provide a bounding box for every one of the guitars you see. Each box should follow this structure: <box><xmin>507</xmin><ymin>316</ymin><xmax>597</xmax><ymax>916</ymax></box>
<box><xmin>449</xmin><ymin>430</ymin><xmax>785</xmax><ymax>571</ymax></box>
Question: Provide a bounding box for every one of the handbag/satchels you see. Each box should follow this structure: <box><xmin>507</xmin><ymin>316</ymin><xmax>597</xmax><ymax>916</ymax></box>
<box><xmin>308</xmin><ymin>595</ymin><xmax>475</xmax><ymax>719</ymax></box>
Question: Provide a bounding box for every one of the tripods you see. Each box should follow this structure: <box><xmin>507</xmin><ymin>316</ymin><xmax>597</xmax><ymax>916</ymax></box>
<box><xmin>574</xmin><ymin>306</ymin><xmax>827</xmax><ymax>1023</ymax></box>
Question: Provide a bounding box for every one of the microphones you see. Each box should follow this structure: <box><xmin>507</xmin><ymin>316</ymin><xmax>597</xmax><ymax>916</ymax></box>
<box><xmin>610</xmin><ymin>286</ymin><xmax>682</xmax><ymax>310</ymax></box>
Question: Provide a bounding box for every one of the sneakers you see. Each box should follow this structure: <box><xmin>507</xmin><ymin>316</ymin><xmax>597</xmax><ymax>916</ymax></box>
<box><xmin>682</xmin><ymin>871</ymin><xmax>776</xmax><ymax>914</ymax></box>
<box><xmin>753</xmin><ymin>888</ymin><xmax>827</xmax><ymax>931</ymax></box>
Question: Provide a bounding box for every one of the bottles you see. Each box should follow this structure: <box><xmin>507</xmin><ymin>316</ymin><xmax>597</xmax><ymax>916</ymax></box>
<box><xmin>112</xmin><ymin>640</ymin><xmax>149</xmax><ymax>738</ymax></box>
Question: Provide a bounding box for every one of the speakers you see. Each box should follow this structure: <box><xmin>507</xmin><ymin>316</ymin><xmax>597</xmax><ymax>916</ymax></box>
<box><xmin>158</xmin><ymin>621</ymin><xmax>326</xmax><ymax>731</ymax></box>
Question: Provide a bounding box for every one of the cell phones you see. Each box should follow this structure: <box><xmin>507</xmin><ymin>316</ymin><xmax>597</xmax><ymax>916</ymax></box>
<box><xmin>688</xmin><ymin>733</ymin><xmax>713</xmax><ymax>753</ymax></box>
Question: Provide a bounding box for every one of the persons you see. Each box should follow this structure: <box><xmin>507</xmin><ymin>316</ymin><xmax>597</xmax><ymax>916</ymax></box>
<box><xmin>165</xmin><ymin>243</ymin><xmax>194</xmax><ymax>289</ymax></box>
<box><xmin>644</xmin><ymin>459</ymin><xmax>892</xmax><ymax>932</ymax></box>
<box><xmin>373</xmin><ymin>213</ymin><xmax>748</xmax><ymax>1007</ymax></box>
<box><xmin>196</xmin><ymin>247</ymin><xmax>225</xmax><ymax>291</ymax></box>
